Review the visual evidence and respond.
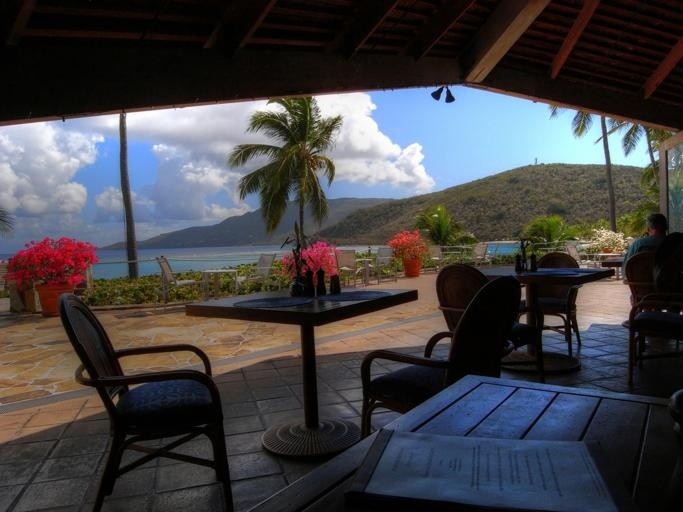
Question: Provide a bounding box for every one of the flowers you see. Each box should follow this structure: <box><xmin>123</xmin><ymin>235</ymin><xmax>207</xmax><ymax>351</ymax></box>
<box><xmin>387</xmin><ymin>228</ymin><xmax>429</xmax><ymax>258</ymax></box>
<box><xmin>2</xmin><ymin>235</ymin><xmax>99</xmax><ymax>290</ymax></box>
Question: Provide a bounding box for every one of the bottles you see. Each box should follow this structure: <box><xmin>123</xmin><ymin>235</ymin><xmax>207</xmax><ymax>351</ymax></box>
<box><xmin>305</xmin><ymin>266</ymin><xmax>315</xmax><ymax>297</ymax></box>
<box><xmin>315</xmin><ymin>266</ymin><xmax>327</xmax><ymax>296</ymax></box>
<box><xmin>530</xmin><ymin>251</ymin><xmax>538</xmax><ymax>272</ymax></box>
<box><xmin>329</xmin><ymin>274</ymin><xmax>341</xmax><ymax>295</ymax></box>
<box><xmin>515</xmin><ymin>252</ymin><xmax>524</xmax><ymax>275</ymax></box>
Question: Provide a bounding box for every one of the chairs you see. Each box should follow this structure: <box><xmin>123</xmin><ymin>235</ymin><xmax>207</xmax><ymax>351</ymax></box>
<box><xmin>58</xmin><ymin>291</ymin><xmax>235</xmax><ymax>511</ymax></box>
<box><xmin>155</xmin><ymin>255</ymin><xmax>197</xmax><ymax>304</ymax></box>
<box><xmin>278</xmin><ymin>241</ymin><xmax>402</xmax><ymax>292</ymax></box>
<box><xmin>359</xmin><ymin>273</ymin><xmax>523</xmax><ymax>441</ymax></box>
<box><xmin>232</xmin><ymin>252</ymin><xmax>276</xmax><ymax>293</ymax></box>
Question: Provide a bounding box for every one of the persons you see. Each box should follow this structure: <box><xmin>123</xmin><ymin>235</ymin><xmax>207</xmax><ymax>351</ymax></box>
<box><xmin>621</xmin><ymin>213</ymin><xmax>669</xmax><ymax>329</ymax></box>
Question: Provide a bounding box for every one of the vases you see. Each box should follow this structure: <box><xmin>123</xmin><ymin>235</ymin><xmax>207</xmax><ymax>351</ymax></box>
<box><xmin>37</xmin><ymin>278</ymin><xmax>75</xmax><ymax>318</ymax></box>
<box><xmin>401</xmin><ymin>255</ymin><xmax>423</xmax><ymax>278</ymax></box>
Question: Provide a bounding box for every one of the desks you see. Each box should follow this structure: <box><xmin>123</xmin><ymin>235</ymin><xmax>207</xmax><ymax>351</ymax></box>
<box><xmin>199</xmin><ymin>269</ymin><xmax>237</xmax><ymax>302</ymax></box>
<box><xmin>248</xmin><ymin>373</ymin><xmax>681</xmax><ymax>512</ymax></box>
<box><xmin>186</xmin><ymin>290</ymin><xmax>418</xmax><ymax>464</ymax></box>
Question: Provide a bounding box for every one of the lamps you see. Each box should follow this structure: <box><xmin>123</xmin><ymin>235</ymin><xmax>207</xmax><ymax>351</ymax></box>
<box><xmin>431</xmin><ymin>86</ymin><xmax>456</xmax><ymax>103</ymax></box>
